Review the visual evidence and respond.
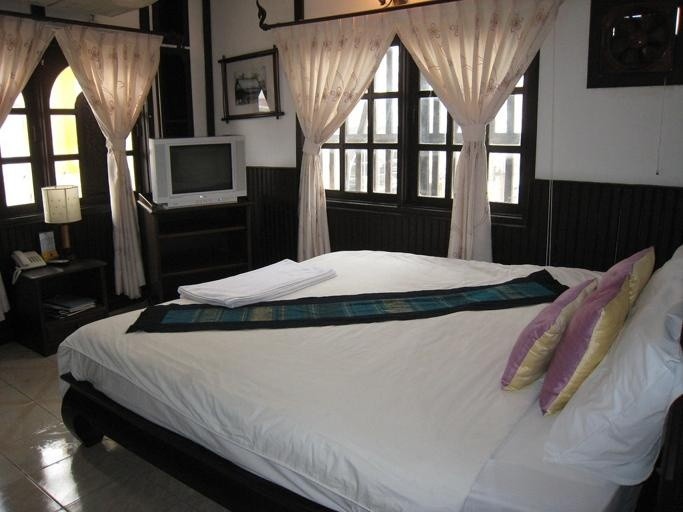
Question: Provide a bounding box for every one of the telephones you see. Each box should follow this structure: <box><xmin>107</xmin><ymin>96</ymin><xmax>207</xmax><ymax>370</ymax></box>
<box><xmin>9</xmin><ymin>248</ymin><xmax>48</xmax><ymax>272</ymax></box>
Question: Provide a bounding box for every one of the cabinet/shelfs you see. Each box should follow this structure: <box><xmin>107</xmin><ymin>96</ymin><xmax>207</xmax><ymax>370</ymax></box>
<box><xmin>136</xmin><ymin>201</ymin><xmax>252</xmax><ymax>303</ymax></box>
<box><xmin>9</xmin><ymin>258</ymin><xmax>110</xmax><ymax>357</ymax></box>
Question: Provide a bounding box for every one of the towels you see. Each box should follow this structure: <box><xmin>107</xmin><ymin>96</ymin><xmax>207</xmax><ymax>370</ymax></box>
<box><xmin>177</xmin><ymin>258</ymin><xmax>339</xmax><ymax>309</ymax></box>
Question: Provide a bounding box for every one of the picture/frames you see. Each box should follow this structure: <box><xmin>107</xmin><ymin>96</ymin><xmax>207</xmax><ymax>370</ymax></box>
<box><xmin>216</xmin><ymin>44</ymin><xmax>284</xmax><ymax>125</ymax></box>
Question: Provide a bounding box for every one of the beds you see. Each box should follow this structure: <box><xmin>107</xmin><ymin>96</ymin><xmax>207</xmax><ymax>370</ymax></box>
<box><xmin>55</xmin><ymin>249</ymin><xmax>683</xmax><ymax>512</ymax></box>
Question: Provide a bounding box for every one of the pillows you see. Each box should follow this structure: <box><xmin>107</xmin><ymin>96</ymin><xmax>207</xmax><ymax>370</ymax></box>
<box><xmin>499</xmin><ymin>245</ymin><xmax>656</xmax><ymax>419</ymax></box>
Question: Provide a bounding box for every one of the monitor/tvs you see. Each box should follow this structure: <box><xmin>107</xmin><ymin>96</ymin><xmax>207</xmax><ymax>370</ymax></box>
<box><xmin>148</xmin><ymin>134</ymin><xmax>248</xmax><ymax>211</ymax></box>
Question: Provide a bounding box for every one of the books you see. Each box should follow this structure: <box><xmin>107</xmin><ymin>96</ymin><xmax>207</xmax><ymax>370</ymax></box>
<box><xmin>42</xmin><ymin>294</ymin><xmax>97</xmax><ymax>321</ymax></box>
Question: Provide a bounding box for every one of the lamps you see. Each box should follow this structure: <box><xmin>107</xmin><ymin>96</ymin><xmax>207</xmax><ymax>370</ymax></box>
<box><xmin>39</xmin><ymin>184</ymin><xmax>82</xmax><ymax>262</ymax></box>
